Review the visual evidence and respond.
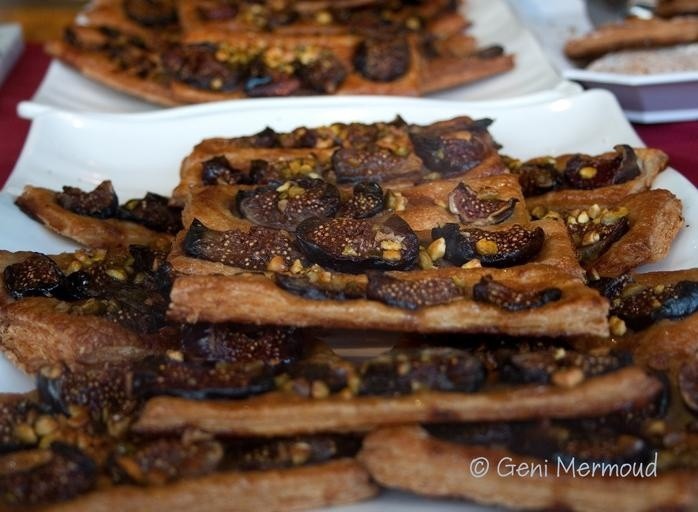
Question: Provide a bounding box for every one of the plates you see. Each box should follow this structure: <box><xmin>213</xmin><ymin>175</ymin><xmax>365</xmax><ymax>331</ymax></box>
<box><xmin>0</xmin><ymin>2</ymin><xmax>698</xmax><ymax>511</ymax></box>
